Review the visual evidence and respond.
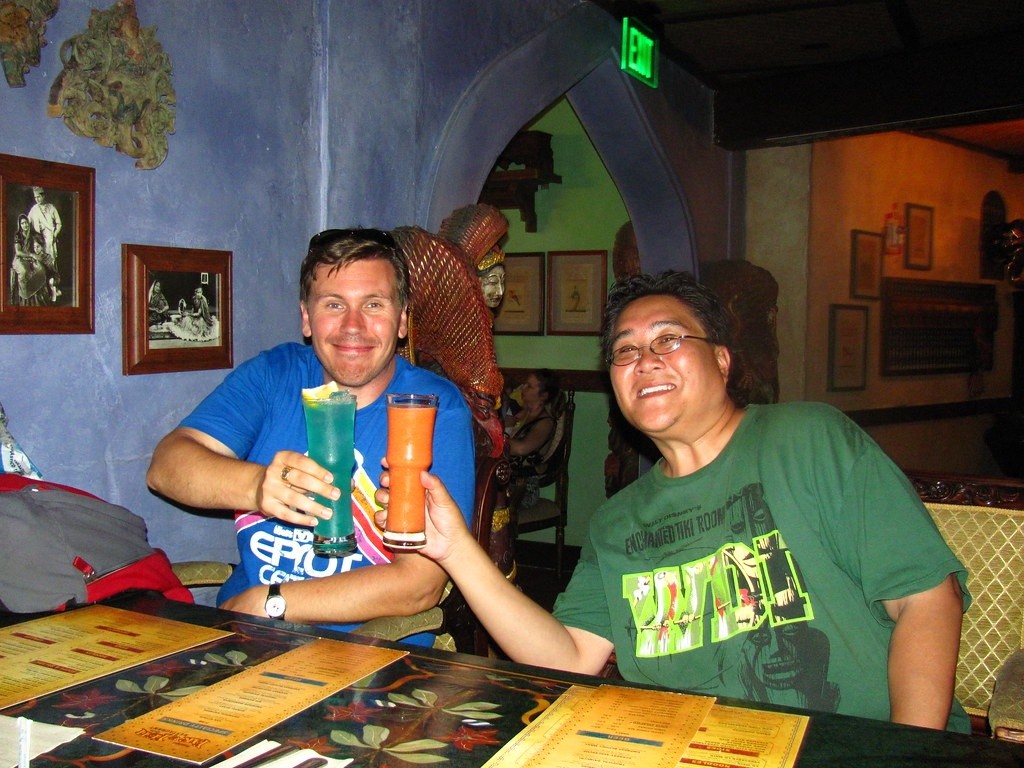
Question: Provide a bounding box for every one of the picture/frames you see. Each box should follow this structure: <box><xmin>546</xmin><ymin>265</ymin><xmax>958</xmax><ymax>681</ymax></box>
<box><xmin>905</xmin><ymin>203</ymin><xmax>936</xmax><ymax>270</ymax></box>
<box><xmin>486</xmin><ymin>252</ymin><xmax>544</xmax><ymax>336</ymax></box>
<box><xmin>849</xmin><ymin>229</ymin><xmax>887</xmax><ymax>300</ymax></box>
<box><xmin>0</xmin><ymin>152</ymin><xmax>97</xmax><ymax>335</ymax></box>
<box><xmin>548</xmin><ymin>249</ymin><xmax>608</xmax><ymax>336</ymax></box>
<box><xmin>122</xmin><ymin>243</ymin><xmax>235</xmax><ymax>375</ymax></box>
<box><xmin>827</xmin><ymin>303</ymin><xmax>868</xmax><ymax>391</ymax></box>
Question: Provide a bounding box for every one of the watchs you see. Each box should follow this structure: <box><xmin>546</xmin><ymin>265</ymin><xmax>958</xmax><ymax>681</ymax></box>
<box><xmin>264</xmin><ymin>583</ymin><xmax>286</xmax><ymax>621</ymax></box>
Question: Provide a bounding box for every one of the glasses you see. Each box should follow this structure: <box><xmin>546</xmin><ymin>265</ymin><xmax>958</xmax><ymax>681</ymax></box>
<box><xmin>604</xmin><ymin>334</ymin><xmax>719</xmax><ymax>367</ymax></box>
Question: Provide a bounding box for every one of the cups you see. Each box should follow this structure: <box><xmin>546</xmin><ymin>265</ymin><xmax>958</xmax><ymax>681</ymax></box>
<box><xmin>382</xmin><ymin>394</ymin><xmax>440</xmax><ymax>549</ymax></box>
<box><xmin>303</xmin><ymin>393</ymin><xmax>357</xmax><ymax>554</ymax></box>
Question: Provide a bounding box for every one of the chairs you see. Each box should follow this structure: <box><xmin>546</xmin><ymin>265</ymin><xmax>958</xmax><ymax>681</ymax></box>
<box><xmin>895</xmin><ymin>476</ymin><xmax>1024</xmax><ymax>748</ymax></box>
<box><xmin>168</xmin><ymin>388</ymin><xmax>514</xmax><ymax>664</ymax></box>
<box><xmin>498</xmin><ymin>387</ymin><xmax>575</xmax><ymax>593</ymax></box>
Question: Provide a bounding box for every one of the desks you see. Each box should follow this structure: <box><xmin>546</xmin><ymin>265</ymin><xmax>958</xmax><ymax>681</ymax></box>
<box><xmin>0</xmin><ymin>602</ymin><xmax>1024</xmax><ymax>768</ymax></box>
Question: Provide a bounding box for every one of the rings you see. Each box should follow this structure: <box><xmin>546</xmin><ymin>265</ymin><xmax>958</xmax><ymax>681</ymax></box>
<box><xmin>280</xmin><ymin>465</ymin><xmax>292</xmax><ymax>482</ymax></box>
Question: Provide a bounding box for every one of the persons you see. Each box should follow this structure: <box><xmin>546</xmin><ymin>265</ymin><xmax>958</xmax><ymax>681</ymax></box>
<box><xmin>148</xmin><ymin>279</ymin><xmax>220</xmax><ymax>342</ymax></box>
<box><xmin>391</xmin><ymin>203</ymin><xmax>523</xmax><ymax>661</ymax></box>
<box><xmin>374</xmin><ymin>268</ymin><xmax>972</xmax><ymax>735</ymax></box>
<box><xmin>145</xmin><ymin>226</ymin><xmax>475</xmax><ymax>648</ymax></box>
<box><xmin>494</xmin><ymin>368</ymin><xmax>558</xmax><ymax>509</ymax></box>
<box><xmin>12</xmin><ymin>185</ymin><xmax>63</xmax><ymax>307</ymax></box>
